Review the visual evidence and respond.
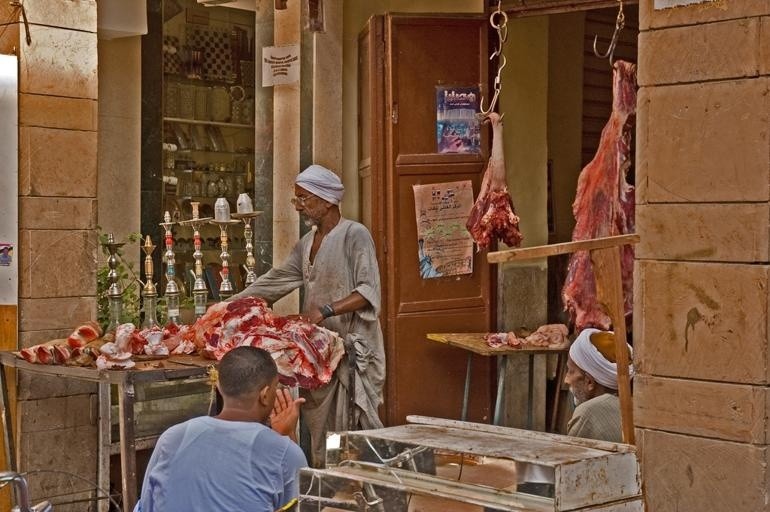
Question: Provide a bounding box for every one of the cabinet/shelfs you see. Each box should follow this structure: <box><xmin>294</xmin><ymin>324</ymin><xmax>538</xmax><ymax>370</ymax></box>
<box><xmin>161</xmin><ymin>27</ymin><xmax>258</xmax><ymax>255</ymax></box>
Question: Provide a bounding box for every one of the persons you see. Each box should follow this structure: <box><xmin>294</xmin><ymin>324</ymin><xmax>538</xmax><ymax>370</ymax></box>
<box><xmin>133</xmin><ymin>346</ymin><xmax>310</xmax><ymax>512</ymax></box>
<box><xmin>201</xmin><ymin>163</ymin><xmax>390</xmax><ymax>468</ymax></box>
<box><xmin>563</xmin><ymin>327</ymin><xmax>636</xmax><ymax>443</ymax></box>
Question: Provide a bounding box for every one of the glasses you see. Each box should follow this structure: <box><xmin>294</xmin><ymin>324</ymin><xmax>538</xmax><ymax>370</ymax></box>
<box><xmin>290</xmin><ymin>195</ymin><xmax>316</xmax><ymax>207</ymax></box>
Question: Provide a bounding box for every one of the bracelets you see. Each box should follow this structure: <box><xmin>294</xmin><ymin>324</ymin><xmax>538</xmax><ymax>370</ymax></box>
<box><xmin>317</xmin><ymin>303</ymin><xmax>335</xmax><ymax>318</ymax></box>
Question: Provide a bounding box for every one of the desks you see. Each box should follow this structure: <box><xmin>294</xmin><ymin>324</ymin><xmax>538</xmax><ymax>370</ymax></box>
<box><xmin>427</xmin><ymin>331</ymin><xmax>572</xmax><ymax>433</ymax></box>
<box><xmin>0</xmin><ymin>336</ymin><xmax>300</xmax><ymax>512</ymax></box>
